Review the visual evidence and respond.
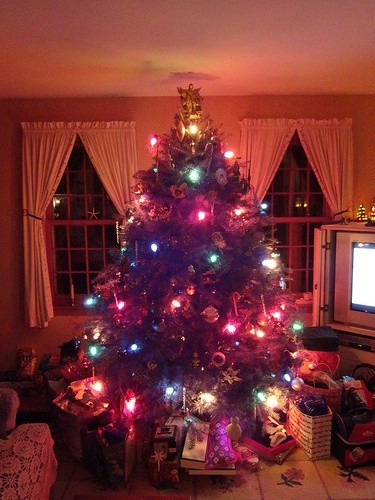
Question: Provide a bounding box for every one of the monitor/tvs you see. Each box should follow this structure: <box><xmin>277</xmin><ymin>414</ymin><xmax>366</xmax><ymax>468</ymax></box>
<box><xmin>333</xmin><ymin>232</ymin><xmax>375</xmax><ymax>329</ymax></box>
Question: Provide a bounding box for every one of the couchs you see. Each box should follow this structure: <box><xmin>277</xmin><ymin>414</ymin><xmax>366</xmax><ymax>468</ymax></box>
<box><xmin>0</xmin><ymin>388</ymin><xmax>58</xmax><ymax>500</ymax></box>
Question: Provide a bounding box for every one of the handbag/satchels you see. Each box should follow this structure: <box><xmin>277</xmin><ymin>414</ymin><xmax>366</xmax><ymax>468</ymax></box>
<box><xmin>285</xmin><ymin>391</ymin><xmax>334</xmax><ymax>462</ymax></box>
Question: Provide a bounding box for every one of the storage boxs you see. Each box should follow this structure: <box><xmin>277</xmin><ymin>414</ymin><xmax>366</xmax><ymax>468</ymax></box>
<box><xmin>334</xmin><ymin>412</ymin><xmax>375</xmax><ymax>468</ymax></box>
<box><xmin>303</xmin><ymin>326</ymin><xmax>340</xmax><ymax>380</ymax></box>
<box><xmin>52</xmin><ymin>392</ymin><xmax>136</xmax><ymax>484</ymax></box>
<box><xmin>152</xmin><ymin>458</ymin><xmax>179</xmax><ymax>488</ymax></box>
<box><xmin>153</xmin><ymin>425</ymin><xmax>178</xmax><ymax>460</ymax></box>
<box><xmin>243</xmin><ymin>436</ymin><xmax>295</xmax><ymax>456</ymax></box>
<box><xmin>182</xmin><ymin>423</ymin><xmax>237</xmax><ymax>476</ymax></box>
